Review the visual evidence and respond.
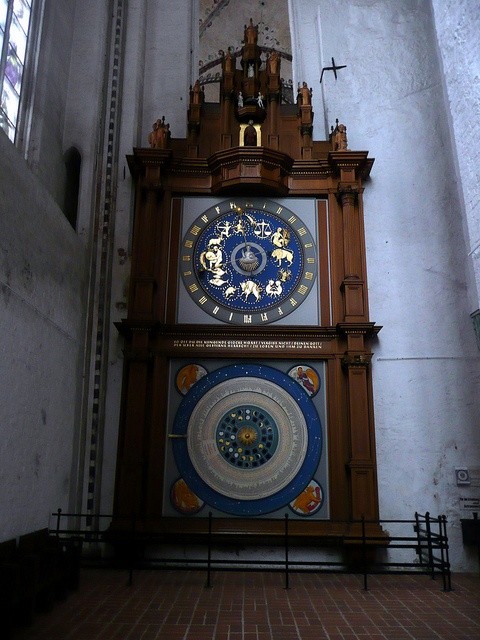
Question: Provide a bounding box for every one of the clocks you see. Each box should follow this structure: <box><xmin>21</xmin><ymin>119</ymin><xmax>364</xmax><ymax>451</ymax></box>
<box><xmin>175</xmin><ymin>194</ymin><xmax>330</xmax><ymax>327</ymax></box>
